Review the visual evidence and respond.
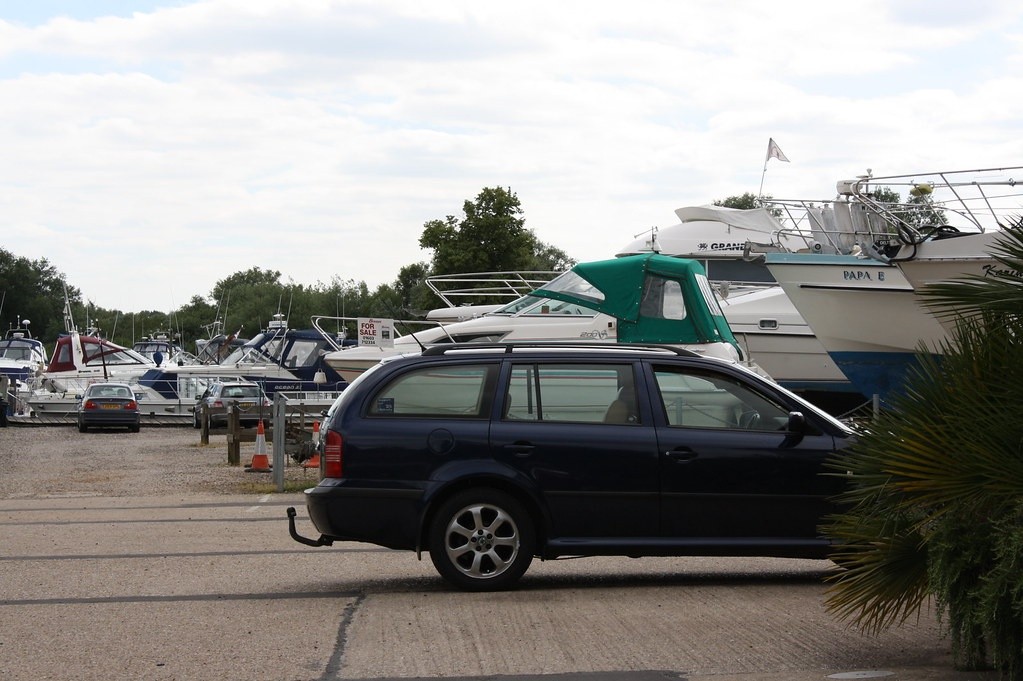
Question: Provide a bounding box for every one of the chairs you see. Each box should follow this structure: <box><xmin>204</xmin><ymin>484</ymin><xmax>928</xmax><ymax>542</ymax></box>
<box><xmin>603</xmin><ymin>387</ymin><xmax>636</xmax><ymax>424</ymax></box>
<box><xmin>92</xmin><ymin>389</ymin><xmax>102</xmax><ymax>396</ymax></box>
<box><xmin>117</xmin><ymin>389</ymin><xmax>127</xmax><ymax>396</ymax></box>
<box><xmin>506</xmin><ymin>394</ymin><xmax>512</xmax><ymax>420</ymax></box>
<box><xmin>242</xmin><ymin>389</ymin><xmax>249</xmax><ymax>394</ymax></box>
<box><xmin>223</xmin><ymin>391</ymin><xmax>230</xmax><ymax>396</ymax></box>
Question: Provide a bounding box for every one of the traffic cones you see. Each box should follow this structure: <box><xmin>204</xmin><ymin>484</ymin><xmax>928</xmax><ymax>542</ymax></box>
<box><xmin>243</xmin><ymin>421</ymin><xmax>273</xmax><ymax>473</ymax></box>
<box><xmin>305</xmin><ymin>422</ymin><xmax>322</xmax><ymax>468</ymax></box>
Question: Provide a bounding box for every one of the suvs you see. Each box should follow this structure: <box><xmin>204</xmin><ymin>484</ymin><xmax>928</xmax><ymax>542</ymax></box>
<box><xmin>286</xmin><ymin>341</ymin><xmax>876</xmax><ymax>593</ymax></box>
<box><xmin>193</xmin><ymin>381</ymin><xmax>271</xmax><ymax>429</ymax></box>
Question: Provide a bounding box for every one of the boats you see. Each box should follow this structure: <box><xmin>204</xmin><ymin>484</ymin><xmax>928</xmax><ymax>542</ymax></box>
<box><xmin>759</xmin><ymin>178</ymin><xmax>1023</xmax><ymax>401</ymax></box>
<box><xmin>0</xmin><ymin>320</ymin><xmax>49</xmax><ymax>406</ymax></box>
<box><xmin>425</xmin><ymin>205</ymin><xmax>858</xmax><ymax>393</ymax></box>
<box><xmin>311</xmin><ymin>253</ymin><xmax>791</xmax><ymax>429</ymax></box>
<box><xmin>24</xmin><ymin>314</ymin><xmax>357</xmax><ymax>416</ymax></box>
<box><xmin>850</xmin><ymin>164</ymin><xmax>1023</xmax><ymax>350</ymax></box>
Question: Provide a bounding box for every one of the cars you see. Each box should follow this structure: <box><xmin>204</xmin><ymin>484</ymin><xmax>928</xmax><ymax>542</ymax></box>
<box><xmin>75</xmin><ymin>383</ymin><xmax>142</xmax><ymax>433</ymax></box>
